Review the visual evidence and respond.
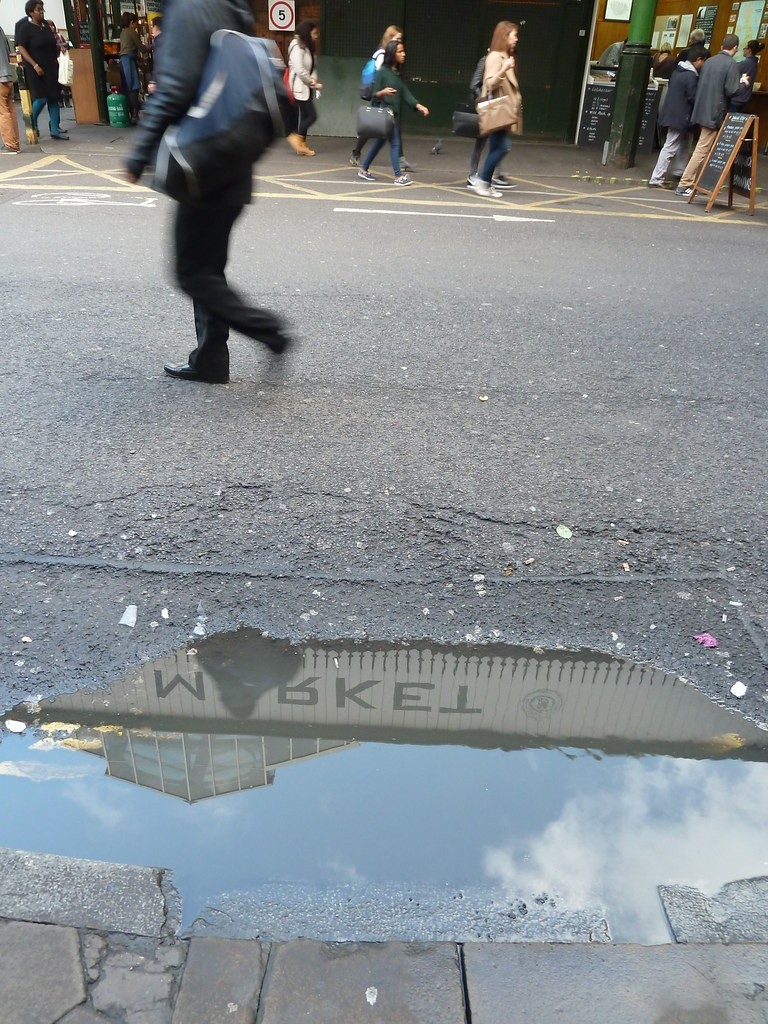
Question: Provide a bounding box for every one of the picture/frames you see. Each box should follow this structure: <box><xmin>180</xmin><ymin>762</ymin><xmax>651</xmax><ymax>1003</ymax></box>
<box><xmin>603</xmin><ymin>0</ymin><xmax>634</xmax><ymax>23</ymax></box>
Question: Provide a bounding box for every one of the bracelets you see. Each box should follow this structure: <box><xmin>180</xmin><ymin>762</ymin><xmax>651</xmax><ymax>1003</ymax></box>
<box><xmin>34</xmin><ymin>64</ymin><xmax>38</xmax><ymax>69</ymax></box>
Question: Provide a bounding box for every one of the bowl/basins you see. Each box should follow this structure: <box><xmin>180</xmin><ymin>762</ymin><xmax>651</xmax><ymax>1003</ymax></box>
<box><xmin>752</xmin><ymin>83</ymin><xmax>761</xmax><ymax>91</ymax></box>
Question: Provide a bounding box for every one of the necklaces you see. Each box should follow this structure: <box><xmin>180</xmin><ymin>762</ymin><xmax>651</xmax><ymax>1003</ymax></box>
<box><xmin>29</xmin><ymin>20</ymin><xmax>43</xmax><ymax>30</ymax></box>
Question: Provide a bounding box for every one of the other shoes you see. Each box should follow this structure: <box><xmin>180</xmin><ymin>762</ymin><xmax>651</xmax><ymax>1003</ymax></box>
<box><xmin>0</xmin><ymin>145</ymin><xmax>21</xmax><ymax>155</ymax></box>
<box><xmin>350</xmin><ymin>150</ymin><xmax>360</xmax><ymax>166</ymax></box>
<box><xmin>648</xmin><ymin>182</ymin><xmax>672</xmax><ymax>189</ymax></box>
<box><xmin>472</xmin><ymin>176</ymin><xmax>502</xmax><ymax>198</ymax></box>
<box><xmin>397</xmin><ymin>157</ymin><xmax>415</xmax><ymax>172</ymax></box>
<box><xmin>56</xmin><ymin>127</ymin><xmax>68</xmax><ymax>133</ymax></box>
<box><xmin>287</xmin><ymin>133</ymin><xmax>314</xmax><ymax>156</ymax></box>
<box><xmin>52</xmin><ymin>133</ymin><xmax>69</xmax><ymax>140</ymax></box>
<box><xmin>466</xmin><ymin>174</ymin><xmax>516</xmax><ymax>190</ymax></box>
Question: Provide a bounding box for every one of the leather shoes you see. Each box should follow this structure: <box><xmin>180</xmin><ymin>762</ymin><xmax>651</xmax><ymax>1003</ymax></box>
<box><xmin>165</xmin><ymin>362</ymin><xmax>209</xmax><ymax>381</ymax></box>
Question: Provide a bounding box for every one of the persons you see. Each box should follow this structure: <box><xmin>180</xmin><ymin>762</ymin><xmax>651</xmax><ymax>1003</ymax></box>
<box><xmin>349</xmin><ymin>25</ymin><xmax>429</xmax><ymax>187</ymax></box>
<box><xmin>675</xmin><ymin>33</ymin><xmax>752</xmax><ymax>196</ymax></box>
<box><xmin>282</xmin><ymin>18</ymin><xmax>325</xmax><ymax>156</ymax></box>
<box><xmin>14</xmin><ymin>0</ymin><xmax>71</xmax><ymax>142</ymax></box>
<box><xmin>599</xmin><ymin>35</ymin><xmax>629</xmax><ymax>66</ymax></box>
<box><xmin>117</xmin><ymin>12</ymin><xmax>154</xmax><ymax>124</ymax></box>
<box><xmin>0</xmin><ymin>25</ymin><xmax>22</xmax><ymax>155</ymax></box>
<box><xmin>676</xmin><ymin>28</ymin><xmax>712</xmax><ymax>63</ymax></box>
<box><xmin>152</xmin><ymin>16</ymin><xmax>163</xmax><ymax>77</ymax></box>
<box><xmin>466</xmin><ymin>21</ymin><xmax>520</xmax><ymax>201</ymax></box>
<box><xmin>649</xmin><ymin>45</ymin><xmax>712</xmax><ymax>189</ymax></box>
<box><xmin>116</xmin><ymin>0</ymin><xmax>292</xmax><ymax>385</ymax></box>
<box><xmin>724</xmin><ymin>40</ymin><xmax>766</xmax><ymax>118</ymax></box>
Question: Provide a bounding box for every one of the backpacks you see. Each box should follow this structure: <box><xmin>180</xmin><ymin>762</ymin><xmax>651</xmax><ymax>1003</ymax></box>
<box><xmin>358</xmin><ymin>52</ymin><xmax>384</xmax><ymax>100</ymax></box>
<box><xmin>283</xmin><ymin>67</ymin><xmax>295</xmax><ymax>104</ymax></box>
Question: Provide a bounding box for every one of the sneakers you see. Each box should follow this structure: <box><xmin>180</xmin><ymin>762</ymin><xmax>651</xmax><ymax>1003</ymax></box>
<box><xmin>358</xmin><ymin>169</ymin><xmax>375</xmax><ymax>180</ymax></box>
<box><xmin>676</xmin><ymin>187</ymin><xmax>697</xmax><ymax>197</ymax></box>
<box><xmin>394</xmin><ymin>174</ymin><xmax>412</xmax><ymax>186</ymax></box>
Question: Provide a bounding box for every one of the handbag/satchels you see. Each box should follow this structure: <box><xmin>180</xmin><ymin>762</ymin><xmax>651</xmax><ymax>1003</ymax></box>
<box><xmin>57</xmin><ymin>50</ymin><xmax>74</xmax><ymax>87</ymax></box>
<box><xmin>452</xmin><ymin>100</ymin><xmax>481</xmax><ymax>138</ymax></box>
<box><xmin>477</xmin><ymin>81</ymin><xmax>518</xmax><ymax>136</ymax></box>
<box><xmin>356</xmin><ymin>93</ymin><xmax>395</xmax><ymax>140</ymax></box>
<box><xmin>152</xmin><ymin>30</ymin><xmax>297</xmax><ymax>210</ymax></box>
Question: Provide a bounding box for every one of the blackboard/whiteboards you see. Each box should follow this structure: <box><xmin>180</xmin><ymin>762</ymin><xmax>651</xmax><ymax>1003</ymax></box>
<box><xmin>696</xmin><ymin>113</ymin><xmax>759</xmax><ymax>197</ymax></box>
<box><xmin>577</xmin><ymin>85</ymin><xmax>660</xmax><ymax>156</ymax></box>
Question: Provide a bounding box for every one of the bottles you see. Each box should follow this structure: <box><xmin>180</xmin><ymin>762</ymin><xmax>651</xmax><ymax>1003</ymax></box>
<box><xmin>15</xmin><ymin>47</ymin><xmax>23</xmax><ymax>66</ymax></box>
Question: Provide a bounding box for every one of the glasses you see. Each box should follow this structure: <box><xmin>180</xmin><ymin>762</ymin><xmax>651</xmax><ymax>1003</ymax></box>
<box><xmin>35</xmin><ymin>9</ymin><xmax>46</xmax><ymax>13</ymax></box>
<box><xmin>743</xmin><ymin>48</ymin><xmax>749</xmax><ymax>50</ymax></box>
